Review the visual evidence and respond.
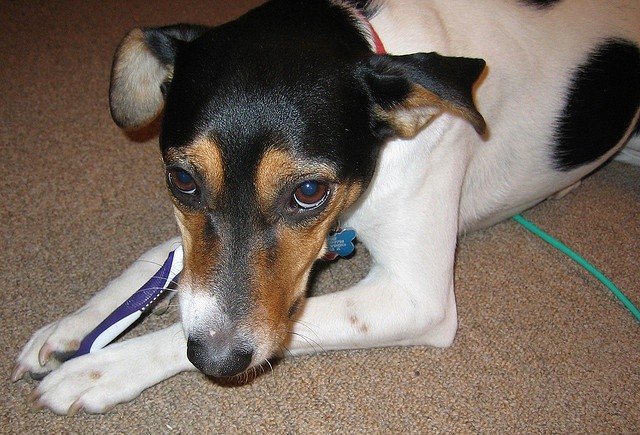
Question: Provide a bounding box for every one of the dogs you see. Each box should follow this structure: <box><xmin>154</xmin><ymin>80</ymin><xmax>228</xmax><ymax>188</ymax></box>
<box><xmin>9</xmin><ymin>0</ymin><xmax>640</xmax><ymax>418</ymax></box>
